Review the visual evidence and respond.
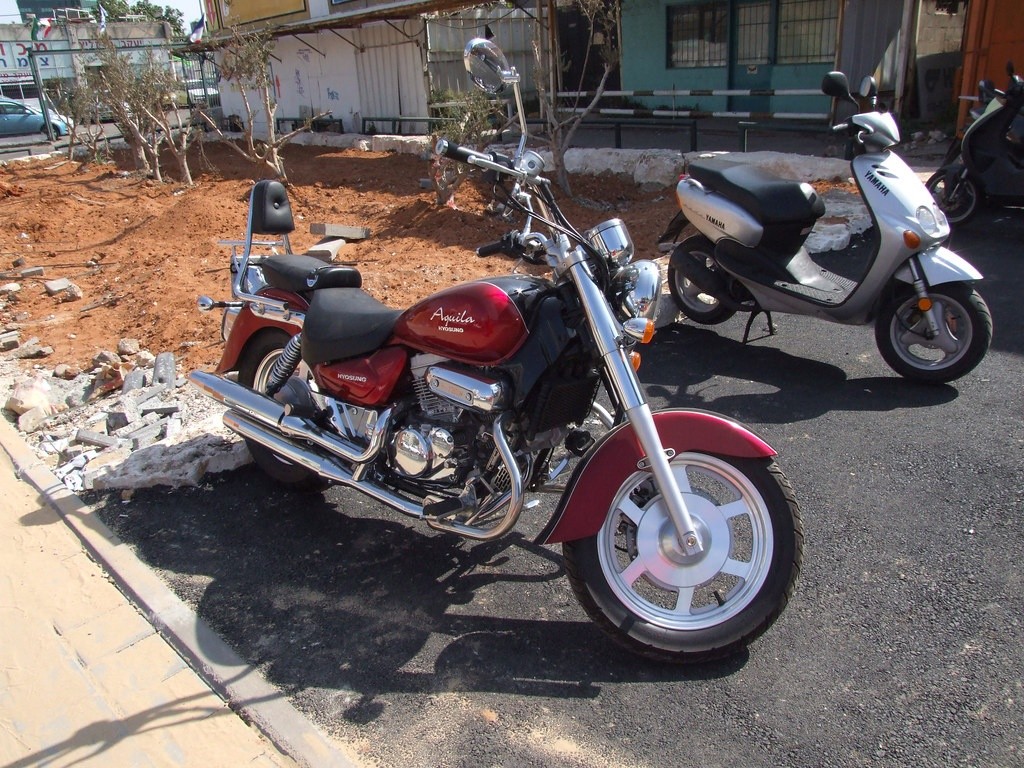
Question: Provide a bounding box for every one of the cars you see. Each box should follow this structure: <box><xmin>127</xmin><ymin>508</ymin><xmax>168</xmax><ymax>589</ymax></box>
<box><xmin>0</xmin><ymin>97</ymin><xmax>74</xmax><ymax>140</ymax></box>
<box><xmin>91</xmin><ymin>78</ymin><xmax>219</xmax><ymax>122</ymax></box>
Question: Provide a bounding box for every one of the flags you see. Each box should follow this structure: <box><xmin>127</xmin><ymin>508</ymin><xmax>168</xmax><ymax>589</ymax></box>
<box><xmin>33</xmin><ymin>16</ymin><xmax>52</xmax><ymax>43</ymax></box>
<box><xmin>186</xmin><ymin>14</ymin><xmax>205</xmax><ymax>44</ymax></box>
<box><xmin>97</xmin><ymin>5</ymin><xmax>109</xmax><ymax>34</ymax></box>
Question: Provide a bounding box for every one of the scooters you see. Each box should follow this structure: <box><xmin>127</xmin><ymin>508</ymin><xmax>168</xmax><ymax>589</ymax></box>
<box><xmin>656</xmin><ymin>71</ymin><xmax>992</xmax><ymax>384</ymax></box>
<box><xmin>923</xmin><ymin>60</ymin><xmax>1024</xmax><ymax>231</ymax></box>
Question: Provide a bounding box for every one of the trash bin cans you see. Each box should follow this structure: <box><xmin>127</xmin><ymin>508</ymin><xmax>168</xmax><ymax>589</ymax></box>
<box><xmin>188</xmin><ymin>88</ymin><xmax>224</xmax><ymax>131</ymax></box>
<box><xmin>47</xmin><ymin>89</ymin><xmax>70</xmax><ymax>115</ymax></box>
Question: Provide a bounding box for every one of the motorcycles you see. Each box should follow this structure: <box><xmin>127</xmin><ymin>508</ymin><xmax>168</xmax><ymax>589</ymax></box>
<box><xmin>184</xmin><ymin>38</ymin><xmax>805</xmax><ymax>666</ymax></box>
